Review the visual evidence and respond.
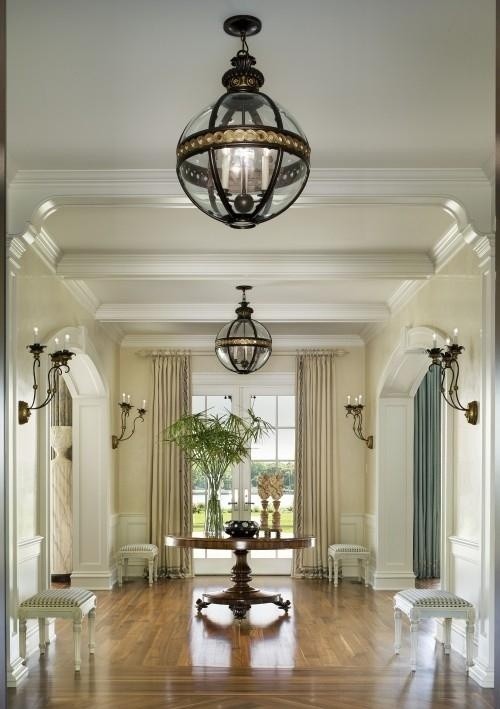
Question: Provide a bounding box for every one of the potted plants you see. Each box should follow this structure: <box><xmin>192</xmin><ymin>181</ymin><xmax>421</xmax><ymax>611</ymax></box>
<box><xmin>161</xmin><ymin>407</ymin><xmax>276</xmax><ymax>537</ymax></box>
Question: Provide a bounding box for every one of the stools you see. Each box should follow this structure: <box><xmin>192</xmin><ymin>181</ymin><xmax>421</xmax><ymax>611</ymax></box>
<box><xmin>327</xmin><ymin>544</ymin><xmax>370</xmax><ymax>588</ymax></box>
<box><xmin>116</xmin><ymin>543</ymin><xmax>159</xmax><ymax>586</ymax></box>
<box><xmin>17</xmin><ymin>589</ymin><xmax>97</xmax><ymax>672</ymax></box>
<box><xmin>392</xmin><ymin>588</ymin><xmax>475</xmax><ymax>673</ymax></box>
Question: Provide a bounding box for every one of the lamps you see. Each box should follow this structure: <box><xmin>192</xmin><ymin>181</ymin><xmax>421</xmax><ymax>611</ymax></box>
<box><xmin>215</xmin><ymin>284</ymin><xmax>272</xmax><ymax>374</ymax></box>
<box><xmin>174</xmin><ymin>12</ymin><xmax>312</xmax><ymax>230</ymax></box>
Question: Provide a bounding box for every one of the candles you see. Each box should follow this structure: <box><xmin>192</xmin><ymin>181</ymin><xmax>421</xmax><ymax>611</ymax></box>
<box><xmin>127</xmin><ymin>395</ymin><xmax>131</xmax><ymax>405</ymax></box>
<box><xmin>355</xmin><ymin>397</ymin><xmax>358</xmax><ymax>405</ymax></box>
<box><xmin>432</xmin><ymin>333</ymin><xmax>437</xmax><ymax>348</ymax></box>
<box><xmin>64</xmin><ymin>335</ymin><xmax>69</xmax><ymax>349</ymax></box>
<box><xmin>445</xmin><ymin>337</ymin><xmax>450</xmax><ymax>351</ymax></box>
<box><xmin>359</xmin><ymin>395</ymin><xmax>362</xmax><ymax>404</ymax></box>
<box><xmin>142</xmin><ymin>400</ymin><xmax>146</xmax><ymax>409</ymax></box>
<box><xmin>55</xmin><ymin>337</ymin><xmax>59</xmax><ymax>352</ymax></box>
<box><xmin>453</xmin><ymin>328</ymin><xmax>459</xmax><ymax>344</ymax></box>
<box><xmin>33</xmin><ymin>328</ymin><xmax>39</xmax><ymax>344</ymax></box>
<box><xmin>348</xmin><ymin>395</ymin><xmax>351</xmax><ymax>404</ymax></box>
<box><xmin>123</xmin><ymin>393</ymin><xmax>126</xmax><ymax>402</ymax></box>
